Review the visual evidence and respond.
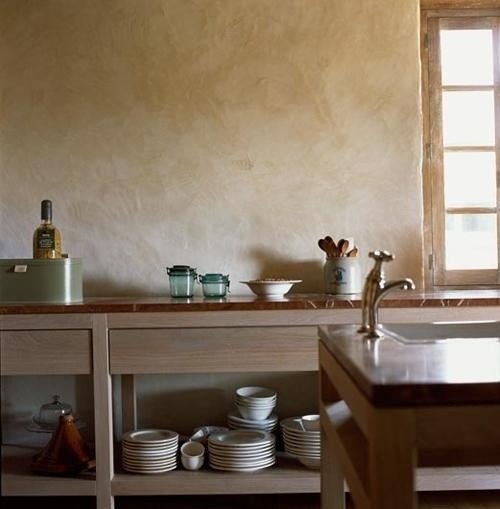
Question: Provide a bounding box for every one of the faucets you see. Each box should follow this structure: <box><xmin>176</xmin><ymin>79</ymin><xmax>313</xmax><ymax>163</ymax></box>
<box><xmin>369</xmin><ymin>277</ymin><xmax>417</xmax><ymax>325</ymax></box>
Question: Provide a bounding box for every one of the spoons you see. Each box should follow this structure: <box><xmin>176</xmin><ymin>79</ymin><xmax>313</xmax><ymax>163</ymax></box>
<box><xmin>318</xmin><ymin>235</ymin><xmax>360</xmax><ymax>258</ymax></box>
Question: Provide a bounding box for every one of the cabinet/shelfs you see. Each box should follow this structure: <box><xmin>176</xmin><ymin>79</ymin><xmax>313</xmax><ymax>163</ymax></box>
<box><xmin>0</xmin><ymin>290</ymin><xmax>500</xmax><ymax>509</ymax></box>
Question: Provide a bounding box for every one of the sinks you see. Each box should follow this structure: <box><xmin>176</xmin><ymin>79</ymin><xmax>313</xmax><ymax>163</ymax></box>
<box><xmin>377</xmin><ymin>318</ymin><xmax>500</xmax><ymax>343</ymax></box>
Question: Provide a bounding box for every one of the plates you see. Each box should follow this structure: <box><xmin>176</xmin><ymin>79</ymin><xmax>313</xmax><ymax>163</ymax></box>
<box><xmin>119</xmin><ymin>383</ymin><xmax>320</xmax><ymax>475</ymax></box>
<box><xmin>23</xmin><ymin>421</ymin><xmax>88</xmax><ymax>434</ymax></box>
<box><xmin>238</xmin><ymin>278</ymin><xmax>303</xmax><ymax>300</ymax></box>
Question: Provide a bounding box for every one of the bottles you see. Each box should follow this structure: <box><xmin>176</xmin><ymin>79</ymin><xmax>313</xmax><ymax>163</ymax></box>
<box><xmin>164</xmin><ymin>264</ymin><xmax>231</xmax><ymax>299</ymax></box>
<box><xmin>32</xmin><ymin>199</ymin><xmax>62</xmax><ymax>260</ymax></box>
